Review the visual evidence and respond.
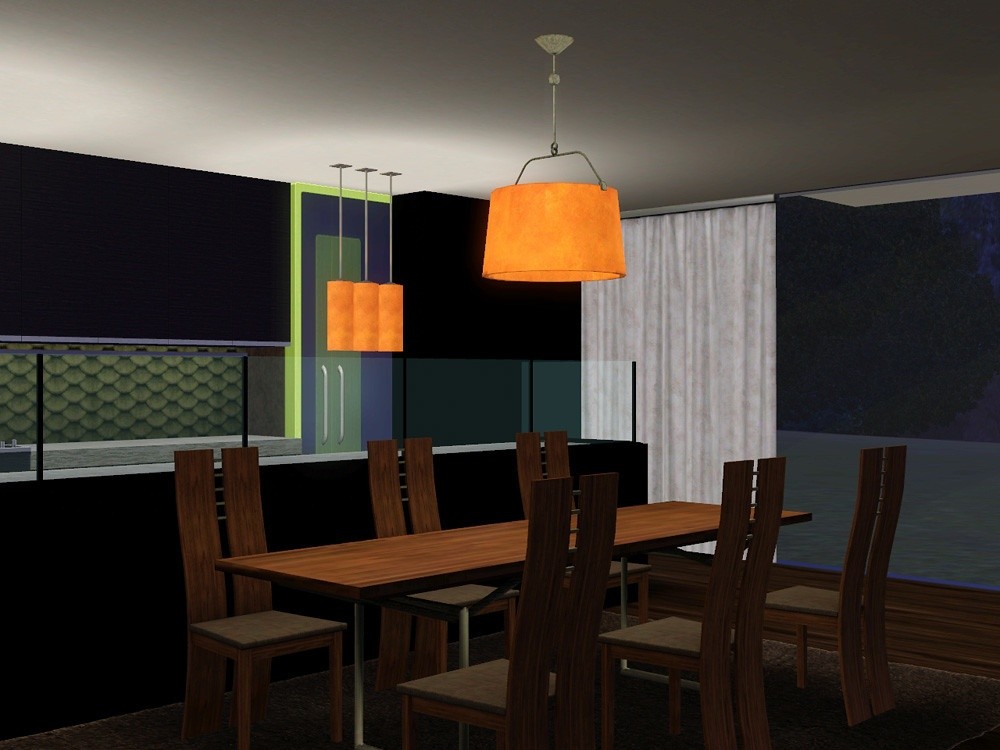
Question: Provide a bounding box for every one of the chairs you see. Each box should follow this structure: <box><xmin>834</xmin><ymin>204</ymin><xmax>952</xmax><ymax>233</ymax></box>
<box><xmin>764</xmin><ymin>444</ymin><xmax>906</xmax><ymax>726</ymax></box>
<box><xmin>367</xmin><ymin>437</ymin><xmax>519</xmax><ymax>692</ymax></box>
<box><xmin>516</xmin><ymin>431</ymin><xmax>651</xmax><ymax>624</ymax></box>
<box><xmin>397</xmin><ymin>472</ymin><xmax>619</xmax><ymax>750</ymax></box>
<box><xmin>597</xmin><ymin>457</ymin><xmax>789</xmax><ymax>750</ymax></box>
<box><xmin>173</xmin><ymin>447</ymin><xmax>349</xmax><ymax>750</ymax></box>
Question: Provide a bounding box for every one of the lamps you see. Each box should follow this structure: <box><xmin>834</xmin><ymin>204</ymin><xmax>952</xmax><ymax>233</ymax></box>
<box><xmin>327</xmin><ymin>164</ymin><xmax>404</xmax><ymax>351</ymax></box>
<box><xmin>482</xmin><ymin>34</ymin><xmax>627</xmax><ymax>282</ymax></box>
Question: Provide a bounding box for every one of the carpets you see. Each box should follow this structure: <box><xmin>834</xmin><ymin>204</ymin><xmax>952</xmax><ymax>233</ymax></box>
<box><xmin>0</xmin><ymin>611</ymin><xmax>1000</xmax><ymax>750</ymax></box>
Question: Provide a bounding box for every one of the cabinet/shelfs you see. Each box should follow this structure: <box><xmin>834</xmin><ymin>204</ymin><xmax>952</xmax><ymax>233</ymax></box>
<box><xmin>246</xmin><ymin>183</ymin><xmax>394</xmax><ymax>453</ymax></box>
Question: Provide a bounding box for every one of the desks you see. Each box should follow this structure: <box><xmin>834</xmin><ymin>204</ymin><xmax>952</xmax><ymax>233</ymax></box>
<box><xmin>216</xmin><ymin>502</ymin><xmax>813</xmax><ymax>750</ymax></box>
